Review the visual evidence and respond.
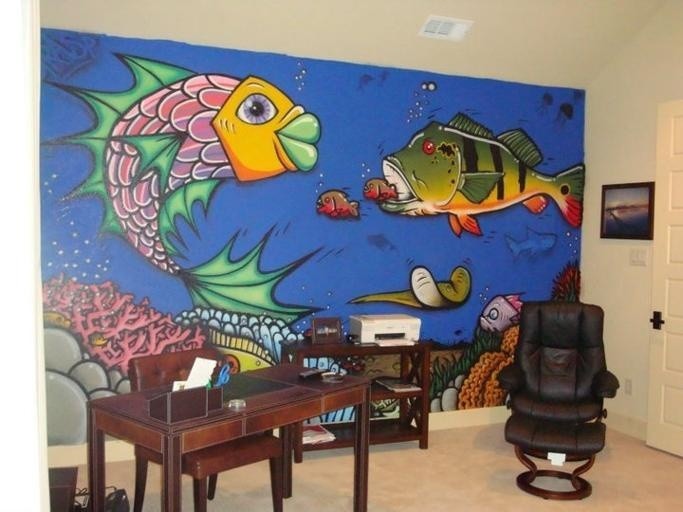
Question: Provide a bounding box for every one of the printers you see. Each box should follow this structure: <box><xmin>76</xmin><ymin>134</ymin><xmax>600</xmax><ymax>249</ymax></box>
<box><xmin>348</xmin><ymin>313</ymin><xmax>422</xmax><ymax>347</ymax></box>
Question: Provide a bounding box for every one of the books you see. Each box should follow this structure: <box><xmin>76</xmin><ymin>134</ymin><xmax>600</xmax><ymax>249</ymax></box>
<box><xmin>375</xmin><ymin>377</ymin><xmax>422</xmax><ymax>392</ymax></box>
<box><xmin>302</xmin><ymin>425</ymin><xmax>336</xmax><ymax>446</ymax></box>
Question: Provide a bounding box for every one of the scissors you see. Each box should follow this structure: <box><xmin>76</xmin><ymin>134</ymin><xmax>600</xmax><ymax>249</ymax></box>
<box><xmin>216</xmin><ymin>363</ymin><xmax>231</xmax><ymax>385</ymax></box>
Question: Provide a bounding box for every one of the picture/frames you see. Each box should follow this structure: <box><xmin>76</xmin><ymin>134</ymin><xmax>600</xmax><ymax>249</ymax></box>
<box><xmin>599</xmin><ymin>182</ymin><xmax>655</xmax><ymax>239</ymax></box>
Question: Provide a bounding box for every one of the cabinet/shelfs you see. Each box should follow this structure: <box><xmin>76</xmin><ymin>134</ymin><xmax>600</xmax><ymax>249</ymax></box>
<box><xmin>279</xmin><ymin>341</ymin><xmax>435</xmax><ymax>461</ymax></box>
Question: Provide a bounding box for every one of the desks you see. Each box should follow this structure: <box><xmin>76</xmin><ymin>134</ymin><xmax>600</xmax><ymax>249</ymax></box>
<box><xmin>84</xmin><ymin>366</ymin><xmax>371</xmax><ymax>511</ymax></box>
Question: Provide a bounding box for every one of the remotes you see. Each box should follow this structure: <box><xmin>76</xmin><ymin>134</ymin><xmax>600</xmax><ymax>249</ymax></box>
<box><xmin>299</xmin><ymin>367</ymin><xmax>330</xmax><ymax>380</ymax></box>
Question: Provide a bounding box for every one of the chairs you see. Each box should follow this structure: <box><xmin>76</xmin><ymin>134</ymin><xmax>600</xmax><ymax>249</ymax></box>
<box><xmin>130</xmin><ymin>350</ymin><xmax>294</xmax><ymax>510</ymax></box>
<box><xmin>497</xmin><ymin>302</ymin><xmax>618</xmax><ymax>500</ymax></box>
